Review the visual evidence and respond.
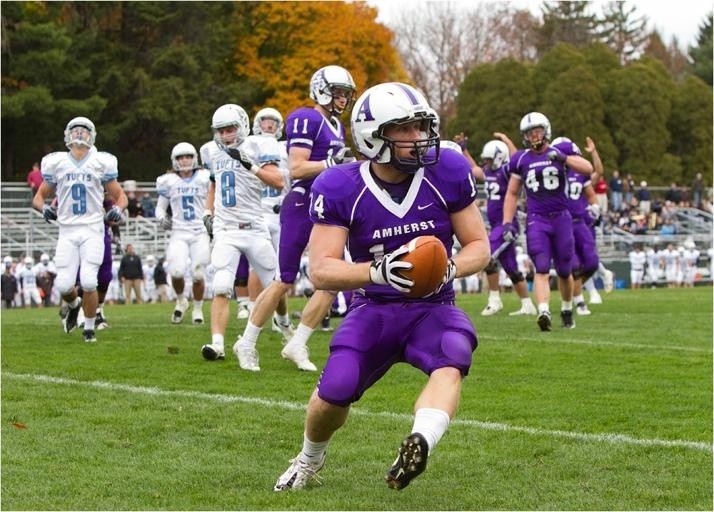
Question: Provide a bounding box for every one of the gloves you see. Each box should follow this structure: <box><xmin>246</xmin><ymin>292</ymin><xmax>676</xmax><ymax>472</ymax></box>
<box><xmin>546</xmin><ymin>150</ymin><xmax>568</xmax><ymax>164</ymax></box>
<box><xmin>105</xmin><ymin>208</ymin><xmax>121</xmax><ymax>222</ymax></box>
<box><xmin>422</xmin><ymin>259</ymin><xmax>456</xmax><ymax>299</ymax></box>
<box><xmin>43</xmin><ymin>206</ymin><xmax>57</xmax><ymax>224</ymax></box>
<box><xmin>327</xmin><ymin>147</ymin><xmax>355</xmax><ymax>168</ymax></box>
<box><xmin>160</xmin><ymin>214</ymin><xmax>172</xmax><ymax>230</ymax></box>
<box><xmin>204</xmin><ymin>215</ymin><xmax>214</xmax><ymax>234</ymax></box>
<box><xmin>370</xmin><ymin>246</ymin><xmax>415</xmax><ymax>293</ymax></box>
<box><xmin>502</xmin><ymin>223</ymin><xmax>518</xmax><ymax>243</ymax></box>
<box><xmin>225</xmin><ymin>147</ymin><xmax>254</xmax><ymax>171</ymax></box>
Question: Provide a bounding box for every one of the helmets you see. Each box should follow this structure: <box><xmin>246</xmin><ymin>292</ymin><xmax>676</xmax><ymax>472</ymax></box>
<box><xmin>171</xmin><ymin>143</ymin><xmax>197</xmax><ymax>172</ymax></box>
<box><xmin>480</xmin><ymin>140</ymin><xmax>509</xmax><ymax>172</ymax></box>
<box><xmin>350</xmin><ymin>82</ymin><xmax>440</xmax><ymax>173</ymax></box>
<box><xmin>253</xmin><ymin>108</ymin><xmax>284</xmax><ymax>140</ymax></box>
<box><xmin>519</xmin><ymin>112</ymin><xmax>551</xmax><ymax>151</ymax></box>
<box><xmin>309</xmin><ymin>65</ymin><xmax>357</xmax><ymax>114</ymax></box>
<box><xmin>64</xmin><ymin>117</ymin><xmax>97</xmax><ymax>148</ymax></box>
<box><xmin>549</xmin><ymin>136</ymin><xmax>581</xmax><ymax>153</ymax></box>
<box><xmin>212</xmin><ymin>104</ymin><xmax>250</xmax><ymax>150</ymax></box>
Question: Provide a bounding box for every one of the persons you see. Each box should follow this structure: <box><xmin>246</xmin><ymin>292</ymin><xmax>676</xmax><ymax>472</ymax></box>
<box><xmin>32</xmin><ymin>117</ymin><xmax>128</xmax><ymax>343</ymax></box>
<box><xmin>78</xmin><ymin>191</ymin><xmax>177</xmax><ymax>304</ymax></box>
<box><xmin>154</xmin><ymin>104</ymin><xmax>353</xmax><ymax>363</ymax></box>
<box><xmin>230</xmin><ymin>63</ymin><xmax>361</xmax><ymax>373</ymax></box>
<box><xmin>595</xmin><ymin>169</ymin><xmax>713</xmax><ymax>288</ymax></box>
<box><xmin>450</xmin><ymin>111</ymin><xmax>614</xmax><ymax>331</ymax></box>
<box><xmin>27</xmin><ymin>164</ymin><xmax>59</xmax><ymax>207</ymax></box>
<box><xmin>272</xmin><ymin>80</ymin><xmax>492</xmax><ymax>496</ymax></box>
<box><xmin>0</xmin><ymin>253</ymin><xmax>66</xmax><ymax>307</ymax></box>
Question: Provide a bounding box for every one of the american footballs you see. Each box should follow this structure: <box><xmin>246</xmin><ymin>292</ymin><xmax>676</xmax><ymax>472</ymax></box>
<box><xmin>395</xmin><ymin>235</ymin><xmax>448</xmax><ymax>299</ymax></box>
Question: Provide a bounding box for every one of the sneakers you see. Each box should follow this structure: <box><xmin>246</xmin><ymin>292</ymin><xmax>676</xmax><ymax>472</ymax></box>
<box><xmin>481</xmin><ymin>270</ymin><xmax>614</xmax><ymax>332</ymax></box>
<box><xmin>60</xmin><ymin>297</ymin><xmax>107</xmax><ymax>342</ymax></box>
<box><xmin>172</xmin><ymin>301</ymin><xmax>333</xmax><ymax>372</ymax></box>
<box><xmin>385</xmin><ymin>432</ymin><xmax>428</xmax><ymax>490</ymax></box>
<box><xmin>274</xmin><ymin>450</ymin><xmax>326</xmax><ymax>492</ymax></box>
